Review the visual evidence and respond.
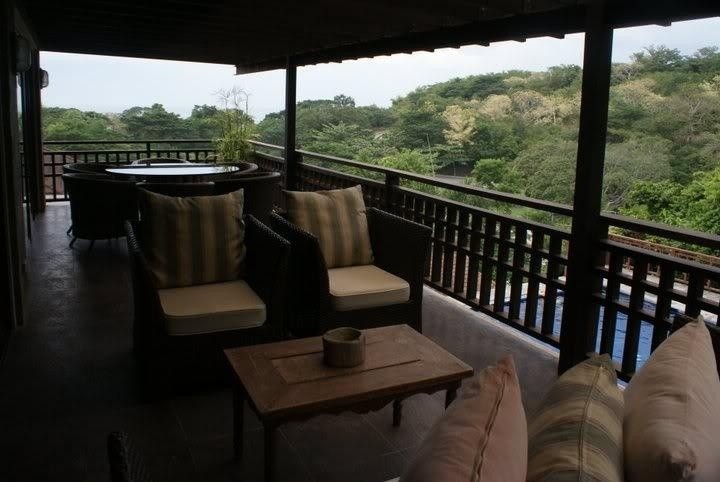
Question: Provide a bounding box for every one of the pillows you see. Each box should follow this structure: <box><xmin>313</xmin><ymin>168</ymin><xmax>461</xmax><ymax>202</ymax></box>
<box><xmin>393</xmin><ymin>347</ymin><xmax>530</xmax><ymax>481</ymax></box>
<box><xmin>523</xmin><ymin>348</ymin><xmax>624</xmax><ymax>481</ymax></box>
<box><xmin>621</xmin><ymin>311</ymin><xmax>719</xmax><ymax>480</ymax></box>
<box><xmin>277</xmin><ymin>183</ymin><xmax>378</xmax><ymax>271</ymax></box>
<box><xmin>132</xmin><ymin>184</ymin><xmax>250</xmax><ymax>288</ymax></box>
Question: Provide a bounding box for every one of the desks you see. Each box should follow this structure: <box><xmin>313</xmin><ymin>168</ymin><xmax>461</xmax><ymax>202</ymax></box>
<box><xmin>219</xmin><ymin>319</ymin><xmax>478</xmax><ymax>480</ymax></box>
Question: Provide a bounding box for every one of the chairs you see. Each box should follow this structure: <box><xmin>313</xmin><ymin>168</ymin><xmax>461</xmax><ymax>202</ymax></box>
<box><xmin>61</xmin><ymin>150</ymin><xmax>284</xmax><ymax>255</ymax></box>
<box><xmin>118</xmin><ymin>206</ymin><xmax>296</xmax><ymax>400</ymax></box>
<box><xmin>271</xmin><ymin>199</ymin><xmax>434</xmax><ymax>336</ymax></box>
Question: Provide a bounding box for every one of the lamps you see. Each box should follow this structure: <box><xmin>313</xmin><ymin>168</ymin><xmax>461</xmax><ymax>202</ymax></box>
<box><xmin>11</xmin><ymin>34</ymin><xmax>33</xmax><ymax>78</ymax></box>
<box><xmin>38</xmin><ymin>64</ymin><xmax>51</xmax><ymax>92</ymax></box>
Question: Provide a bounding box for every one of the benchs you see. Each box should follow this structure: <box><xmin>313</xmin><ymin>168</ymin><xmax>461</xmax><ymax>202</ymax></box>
<box><xmin>104</xmin><ymin>311</ymin><xmax>719</xmax><ymax>481</ymax></box>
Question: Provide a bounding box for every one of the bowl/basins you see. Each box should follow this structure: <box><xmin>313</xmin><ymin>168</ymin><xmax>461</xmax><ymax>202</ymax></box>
<box><xmin>321</xmin><ymin>327</ymin><xmax>366</xmax><ymax>367</ymax></box>
